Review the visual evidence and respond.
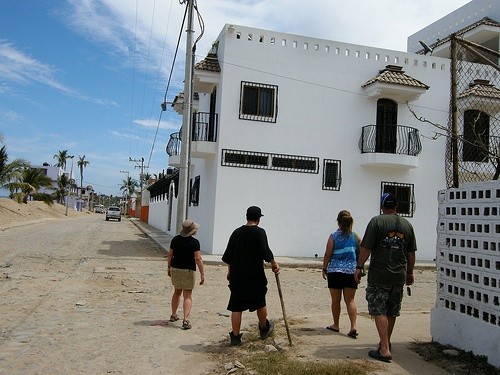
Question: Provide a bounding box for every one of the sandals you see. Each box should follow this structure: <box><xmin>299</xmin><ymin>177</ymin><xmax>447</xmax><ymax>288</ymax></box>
<box><xmin>170</xmin><ymin>314</ymin><xmax>180</xmax><ymax>322</ymax></box>
<box><xmin>181</xmin><ymin>320</ymin><xmax>191</xmax><ymax>329</ymax></box>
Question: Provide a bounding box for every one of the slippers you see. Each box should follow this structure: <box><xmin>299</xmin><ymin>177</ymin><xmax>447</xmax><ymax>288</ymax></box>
<box><xmin>326</xmin><ymin>325</ymin><xmax>339</xmax><ymax>332</ymax></box>
<box><xmin>347</xmin><ymin>330</ymin><xmax>359</xmax><ymax>337</ymax></box>
<box><xmin>368</xmin><ymin>347</ymin><xmax>393</xmax><ymax>361</ymax></box>
<box><xmin>379</xmin><ymin>339</ymin><xmax>391</xmax><ymax>350</ymax></box>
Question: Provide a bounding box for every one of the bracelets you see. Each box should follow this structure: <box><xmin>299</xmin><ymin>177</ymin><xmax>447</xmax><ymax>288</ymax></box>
<box><xmin>356</xmin><ymin>266</ymin><xmax>363</xmax><ymax>269</ymax></box>
<box><xmin>406</xmin><ymin>271</ymin><xmax>413</xmax><ymax>274</ymax></box>
<box><xmin>168</xmin><ymin>265</ymin><xmax>171</xmax><ymax>268</ymax></box>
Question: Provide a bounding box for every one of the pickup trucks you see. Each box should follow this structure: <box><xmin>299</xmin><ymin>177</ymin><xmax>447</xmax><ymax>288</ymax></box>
<box><xmin>106</xmin><ymin>207</ymin><xmax>122</xmax><ymax>222</ymax></box>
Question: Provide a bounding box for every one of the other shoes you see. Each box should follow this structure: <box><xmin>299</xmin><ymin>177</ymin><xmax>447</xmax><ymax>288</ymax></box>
<box><xmin>229</xmin><ymin>330</ymin><xmax>243</xmax><ymax>346</ymax></box>
<box><xmin>258</xmin><ymin>318</ymin><xmax>274</xmax><ymax>339</ymax></box>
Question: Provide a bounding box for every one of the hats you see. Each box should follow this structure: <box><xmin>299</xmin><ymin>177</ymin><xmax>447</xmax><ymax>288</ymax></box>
<box><xmin>180</xmin><ymin>219</ymin><xmax>200</xmax><ymax>238</ymax></box>
<box><xmin>246</xmin><ymin>206</ymin><xmax>264</xmax><ymax>218</ymax></box>
<box><xmin>380</xmin><ymin>193</ymin><xmax>399</xmax><ymax>207</ymax></box>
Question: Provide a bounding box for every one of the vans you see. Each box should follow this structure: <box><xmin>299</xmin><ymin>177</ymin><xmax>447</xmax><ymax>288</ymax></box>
<box><xmin>96</xmin><ymin>205</ymin><xmax>105</xmax><ymax>213</ymax></box>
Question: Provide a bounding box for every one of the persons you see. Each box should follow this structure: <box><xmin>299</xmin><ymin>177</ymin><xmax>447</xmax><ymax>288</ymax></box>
<box><xmin>167</xmin><ymin>219</ymin><xmax>204</xmax><ymax>330</ymax></box>
<box><xmin>322</xmin><ymin>210</ymin><xmax>362</xmax><ymax>337</ymax></box>
<box><xmin>354</xmin><ymin>193</ymin><xmax>417</xmax><ymax>362</ymax></box>
<box><xmin>221</xmin><ymin>206</ymin><xmax>280</xmax><ymax>347</ymax></box>
